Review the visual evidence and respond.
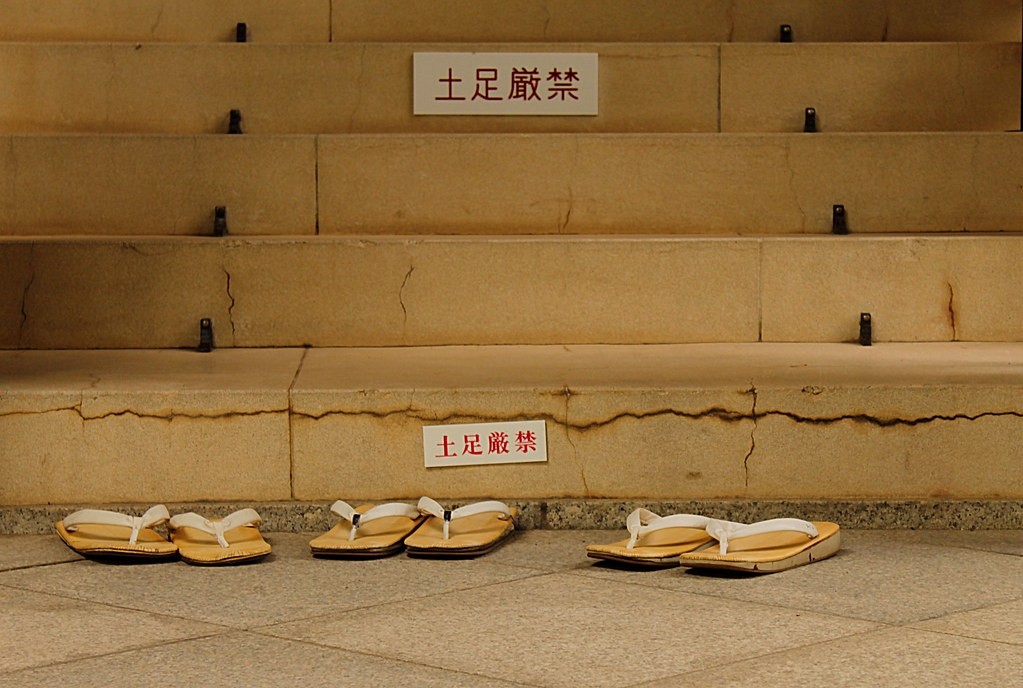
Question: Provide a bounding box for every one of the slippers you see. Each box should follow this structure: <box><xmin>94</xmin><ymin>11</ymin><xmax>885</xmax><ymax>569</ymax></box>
<box><xmin>306</xmin><ymin>496</ymin><xmax>518</xmax><ymax>560</ymax></box>
<box><xmin>53</xmin><ymin>503</ymin><xmax>272</xmax><ymax>565</ymax></box>
<box><xmin>586</xmin><ymin>507</ymin><xmax>842</xmax><ymax>574</ymax></box>
